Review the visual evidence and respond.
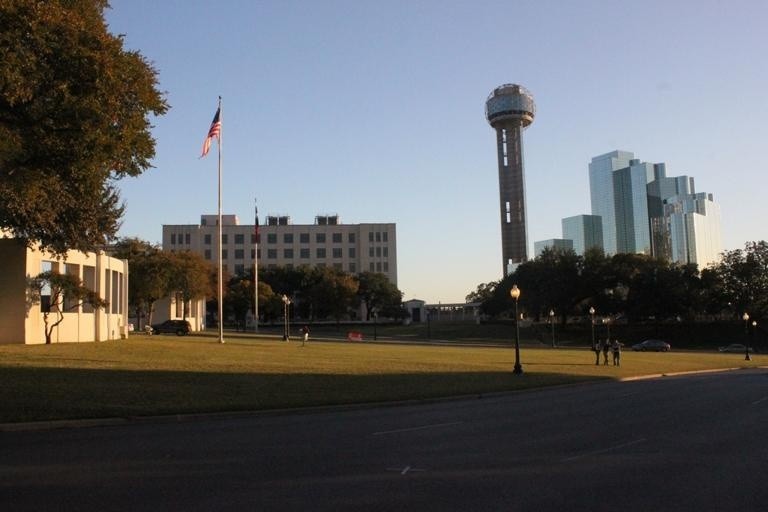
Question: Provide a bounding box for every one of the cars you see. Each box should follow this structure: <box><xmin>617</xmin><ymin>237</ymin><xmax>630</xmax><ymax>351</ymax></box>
<box><xmin>719</xmin><ymin>343</ymin><xmax>752</xmax><ymax>353</ymax></box>
<box><xmin>596</xmin><ymin>315</ymin><xmax>627</xmax><ymax>324</ymax></box>
<box><xmin>206</xmin><ymin>318</ymin><xmax>245</xmax><ymax>328</ymax></box>
<box><xmin>525</xmin><ymin>314</ymin><xmax>588</xmax><ymax>325</ymax></box>
<box><xmin>632</xmin><ymin>339</ymin><xmax>670</xmax><ymax>352</ymax></box>
<box><xmin>128</xmin><ymin>323</ymin><xmax>134</xmax><ymax>332</ymax></box>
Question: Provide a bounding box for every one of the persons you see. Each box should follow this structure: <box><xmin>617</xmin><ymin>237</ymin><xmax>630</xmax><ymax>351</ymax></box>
<box><xmin>611</xmin><ymin>338</ymin><xmax>625</xmax><ymax>367</ymax></box>
<box><xmin>594</xmin><ymin>339</ymin><xmax>602</xmax><ymax>366</ymax></box>
<box><xmin>301</xmin><ymin>325</ymin><xmax>311</xmax><ymax>346</ymax></box>
<box><xmin>602</xmin><ymin>337</ymin><xmax>612</xmax><ymax>366</ymax></box>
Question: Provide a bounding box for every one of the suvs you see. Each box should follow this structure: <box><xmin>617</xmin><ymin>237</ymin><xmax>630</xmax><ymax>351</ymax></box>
<box><xmin>151</xmin><ymin>320</ymin><xmax>192</xmax><ymax>336</ymax></box>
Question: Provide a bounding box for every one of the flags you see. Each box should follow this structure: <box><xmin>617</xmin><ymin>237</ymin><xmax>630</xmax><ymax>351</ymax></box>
<box><xmin>254</xmin><ymin>206</ymin><xmax>261</xmax><ymax>243</ymax></box>
<box><xmin>197</xmin><ymin>105</ymin><xmax>220</xmax><ymax>157</ymax></box>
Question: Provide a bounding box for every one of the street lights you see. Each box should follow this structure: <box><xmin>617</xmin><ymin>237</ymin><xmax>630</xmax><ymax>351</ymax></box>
<box><xmin>549</xmin><ymin>309</ymin><xmax>555</xmax><ymax>346</ymax></box>
<box><xmin>286</xmin><ymin>299</ymin><xmax>291</xmax><ymax>338</ymax></box>
<box><xmin>751</xmin><ymin>321</ymin><xmax>758</xmax><ymax>353</ymax></box>
<box><xmin>373</xmin><ymin>311</ymin><xmax>377</xmax><ymax>341</ymax></box>
<box><xmin>282</xmin><ymin>294</ymin><xmax>288</xmax><ymax>341</ymax></box>
<box><xmin>742</xmin><ymin>312</ymin><xmax>750</xmax><ymax>361</ymax></box>
<box><xmin>425</xmin><ymin>306</ymin><xmax>431</xmax><ymax>340</ymax></box>
<box><xmin>509</xmin><ymin>285</ymin><xmax>524</xmax><ymax>374</ymax></box>
<box><xmin>588</xmin><ymin>307</ymin><xmax>596</xmax><ymax>350</ymax></box>
<box><xmin>605</xmin><ymin>314</ymin><xmax>611</xmax><ymax>346</ymax></box>
